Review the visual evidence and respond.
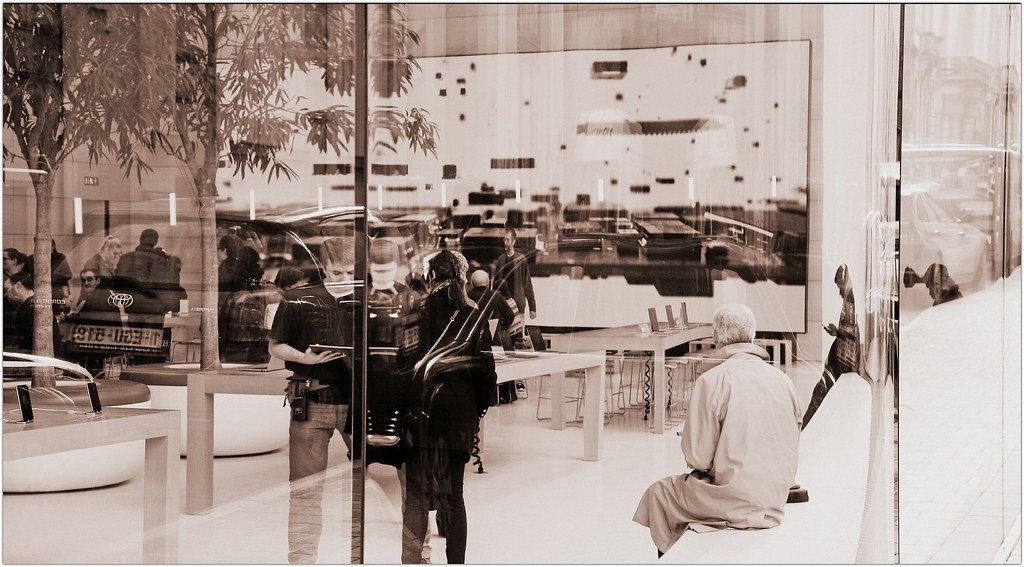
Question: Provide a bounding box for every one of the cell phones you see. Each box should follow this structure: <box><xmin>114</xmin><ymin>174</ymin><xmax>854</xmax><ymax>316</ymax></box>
<box><xmin>822</xmin><ymin>323</ymin><xmax>832</xmax><ymax>333</ymax></box>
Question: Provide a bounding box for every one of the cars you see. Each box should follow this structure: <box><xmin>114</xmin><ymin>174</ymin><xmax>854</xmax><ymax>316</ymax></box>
<box><xmin>4</xmin><ymin>202</ymin><xmax>388</xmax><ymax>352</ymax></box>
<box><xmin>897</xmin><ymin>141</ymin><xmax>1020</xmax><ymax>307</ymax></box>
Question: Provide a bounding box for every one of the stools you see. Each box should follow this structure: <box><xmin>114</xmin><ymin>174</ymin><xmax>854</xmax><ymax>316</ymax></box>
<box><xmin>580</xmin><ymin>352</ymin><xmax>706</xmax><ymax>430</ymax></box>
<box><xmin>171</xmin><ymin>338</ymin><xmax>203</xmax><ymax>364</ymax></box>
<box><xmin>86</xmin><ymin>353</ymin><xmax>128</xmax><ymax>380</ymax></box>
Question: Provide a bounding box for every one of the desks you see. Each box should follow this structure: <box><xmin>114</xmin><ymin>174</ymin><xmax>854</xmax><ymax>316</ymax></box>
<box><xmin>186</xmin><ymin>366</ymin><xmax>292</xmax><ymax>516</ymax></box>
<box><xmin>490</xmin><ymin>348</ymin><xmax>607</xmax><ymax>461</ymax></box>
<box><xmin>2</xmin><ymin>404</ymin><xmax>181</xmax><ymax>564</ymax></box>
<box><xmin>247</xmin><ymin>204</ymin><xmax>807</xmax><ymax>297</ymax></box>
<box><xmin>164</xmin><ymin>309</ymin><xmax>202</xmax><ymax>362</ymax></box>
<box><xmin>550</xmin><ymin>321</ymin><xmax>714</xmax><ymax>435</ymax></box>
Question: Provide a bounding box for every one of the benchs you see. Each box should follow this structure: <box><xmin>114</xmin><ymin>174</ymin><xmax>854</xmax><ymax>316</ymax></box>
<box><xmin>123</xmin><ymin>361</ymin><xmax>295</xmax><ymax>456</ymax></box>
<box><xmin>690</xmin><ymin>338</ymin><xmax>792</xmax><ymax>371</ymax></box>
<box><xmin>3</xmin><ymin>378</ymin><xmax>149</xmax><ymax>491</ymax></box>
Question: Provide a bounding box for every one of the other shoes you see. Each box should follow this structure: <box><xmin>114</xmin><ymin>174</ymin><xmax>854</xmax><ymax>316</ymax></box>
<box><xmin>516</xmin><ymin>382</ymin><xmax>527</xmax><ymax>399</ymax></box>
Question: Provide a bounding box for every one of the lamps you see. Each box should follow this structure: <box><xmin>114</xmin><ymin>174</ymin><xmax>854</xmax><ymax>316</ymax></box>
<box><xmin>592</xmin><ymin>61</ymin><xmax>628</xmax><ymax>79</ymax></box>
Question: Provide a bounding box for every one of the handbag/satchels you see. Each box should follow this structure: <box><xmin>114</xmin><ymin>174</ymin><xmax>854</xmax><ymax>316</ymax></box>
<box><xmin>412</xmin><ymin>354</ymin><xmax>491</xmax><ymax>407</ymax></box>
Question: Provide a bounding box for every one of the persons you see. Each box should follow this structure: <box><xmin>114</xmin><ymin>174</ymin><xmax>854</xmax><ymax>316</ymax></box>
<box><xmin>801</xmin><ymin>263</ymin><xmax>861</xmax><ymax>432</ymax></box>
<box><xmin>489</xmin><ymin>229</ymin><xmax>537</xmax><ymax>391</ymax></box>
<box><xmin>218</xmin><ymin>237</ymin><xmax>358</xmax><ymax>564</ymax></box>
<box><xmin>924</xmin><ymin>264</ymin><xmax>964</xmax><ymax>308</ymax></box>
<box><xmin>633</xmin><ymin>305</ymin><xmax>803</xmax><ymax>559</ymax></box>
<box><xmin>493</xmin><ymin>279</ymin><xmax>521</xmax><ymax>336</ymax></box>
<box><xmin>365</xmin><ymin>240</ymin><xmax>411</xmax><ymax>406</ymax></box>
<box><xmin>3</xmin><ymin>228</ymin><xmax>188</xmax><ymax>370</ymax></box>
<box><xmin>365</xmin><ymin>249</ymin><xmax>497</xmax><ymax>564</ymax></box>
<box><xmin>468</xmin><ymin>270</ymin><xmax>515</xmax><ymax>346</ymax></box>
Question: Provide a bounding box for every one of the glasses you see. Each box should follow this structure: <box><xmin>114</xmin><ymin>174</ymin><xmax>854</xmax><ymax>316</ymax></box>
<box><xmin>80</xmin><ymin>276</ymin><xmax>94</xmax><ymax>283</ymax></box>
<box><xmin>3</xmin><ymin>256</ymin><xmax>12</xmax><ymax>261</ymax></box>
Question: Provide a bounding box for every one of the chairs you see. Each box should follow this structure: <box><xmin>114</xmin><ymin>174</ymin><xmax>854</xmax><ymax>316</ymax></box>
<box><xmin>486</xmin><ymin>303</ymin><xmax>714</xmax><ymax>431</ymax></box>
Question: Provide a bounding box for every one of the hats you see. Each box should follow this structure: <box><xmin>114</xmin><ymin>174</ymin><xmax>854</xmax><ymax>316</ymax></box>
<box><xmin>140</xmin><ymin>229</ymin><xmax>158</xmax><ymax>245</ymax></box>
<box><xmin>217</xmin><ymin>235</ymin><xmax>235</xmax><ymax>249</ymax></box>
